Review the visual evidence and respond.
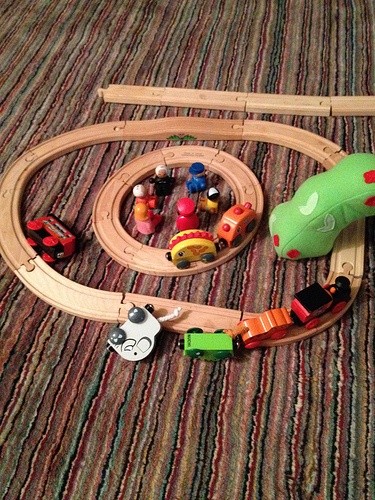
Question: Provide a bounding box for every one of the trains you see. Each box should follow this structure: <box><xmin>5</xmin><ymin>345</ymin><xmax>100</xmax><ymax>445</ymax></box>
<box><xmin>177</xmin><ymin>275</ymin><xmax>351</xmax><ymax>362</ymax></box>
<box><xmin>166</xmin><ymin>202</ymin><xmax>256</xmax><ymax>269</ymax></box>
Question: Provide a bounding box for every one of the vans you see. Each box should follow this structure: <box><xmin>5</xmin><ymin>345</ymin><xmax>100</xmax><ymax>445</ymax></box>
<box><xmin>26</xmin><ymin>213</ymin><xmax>77</xmax><ymax>264</ymax></box>
